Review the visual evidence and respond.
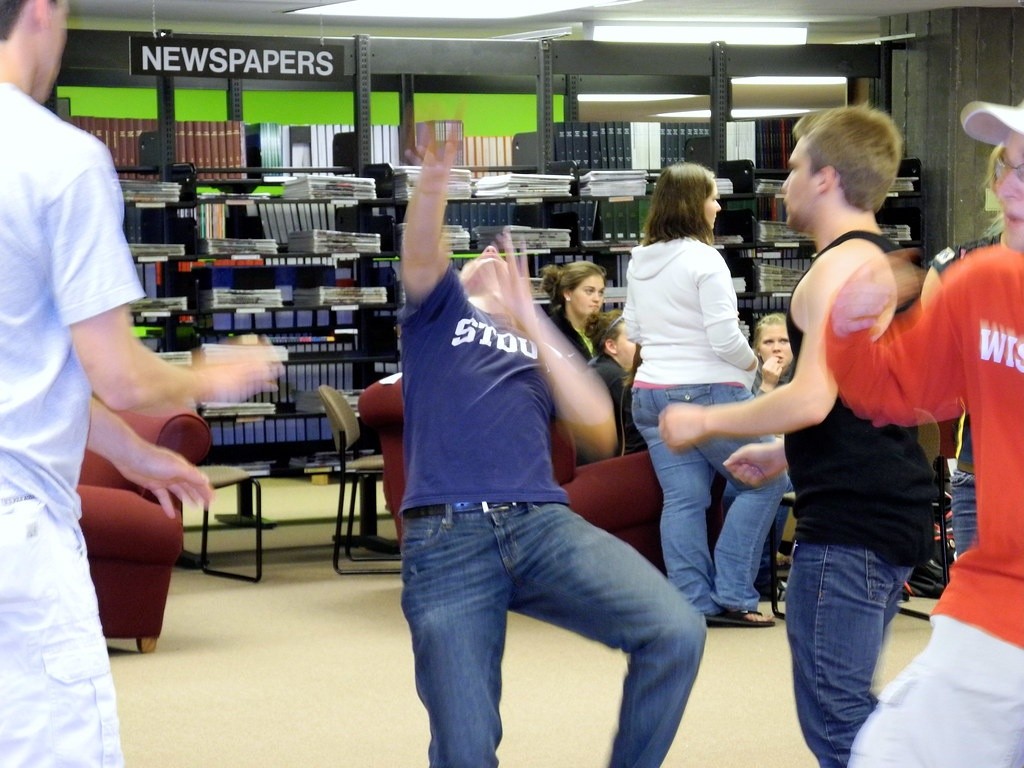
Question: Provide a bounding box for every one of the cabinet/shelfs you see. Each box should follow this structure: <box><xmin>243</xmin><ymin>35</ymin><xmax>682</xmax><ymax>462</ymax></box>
<box><xmin>118</xmin><ymin>160</ymin><xmax>920</xmax><ymax>480</ymax></box>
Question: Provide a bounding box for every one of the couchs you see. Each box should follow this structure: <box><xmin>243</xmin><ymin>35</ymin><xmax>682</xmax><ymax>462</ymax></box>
<box><xmin>76</xmin><ymin>393</ymin><xmax>212</xmax><ymax>652</ymax></box>
<box><xmin>359</xmin><ymin>375</ymin><xmax>725</xmax><ymax>577</ymax></box>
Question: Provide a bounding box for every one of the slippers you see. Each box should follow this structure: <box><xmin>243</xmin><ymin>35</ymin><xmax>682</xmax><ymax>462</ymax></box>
<box><xmin>704</xmin><ymin>607</ymin><xmax>775</xmax><ymax>628</ymax></box>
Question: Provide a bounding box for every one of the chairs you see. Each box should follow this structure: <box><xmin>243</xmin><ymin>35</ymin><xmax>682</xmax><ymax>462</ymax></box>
<box><xmin>768</xmin><ymin>409</ymin><xmax>949</xmax><ymax>622</ymax></box>
<box><xmin>317</xmin><ymin>383</ymin><xmax>405</xmax><ymax>575</ymax></box>
<box><xmin>176</xmin><ymin>467</ymin><xmax>263</xmax><ymax>581</ymax></box>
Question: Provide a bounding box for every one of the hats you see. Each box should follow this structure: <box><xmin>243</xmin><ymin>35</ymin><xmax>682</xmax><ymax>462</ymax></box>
<box><xmin>962</xmin><ymin>99</ymin><xmax>1024</xmax><ymax>144</ymax></box>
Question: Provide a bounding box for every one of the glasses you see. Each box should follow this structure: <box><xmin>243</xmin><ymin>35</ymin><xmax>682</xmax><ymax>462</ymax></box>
<box><xmin>992</xmin><ymin>157</ymin><xmax>1024</xmax><ymax>182</ymax></box>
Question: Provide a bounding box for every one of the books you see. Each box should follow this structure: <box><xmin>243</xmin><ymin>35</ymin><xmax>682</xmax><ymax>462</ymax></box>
<box><xmin>711</xmin><ymin>115</ymin><xmax>818</xmax><ymax>344</ymax></box>
<box><xmin>877</xmin><ymin>175</ymin><xmax>921</xmax><ymax>245</ymax></box>
<box><xmin>70</xmin><ymin>114</ymin><xmax>403</xmax><ymax>478</ymax></box>
<box><xmin>396</xmin><ymin>121</ymin><xmax>656</xmax><ymax>312</ymax></box>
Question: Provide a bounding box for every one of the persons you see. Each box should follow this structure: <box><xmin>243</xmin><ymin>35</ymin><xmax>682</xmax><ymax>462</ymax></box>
<box><xmin>915</xmin><ymin>143</ymin><xmax>1006</xmax><ymax>561</ymax></box>
<box><xmin>657</xmin><ymin>99</ymin><xmax>939</xmax><ymax>768</ymax></box>
<box><xmin>577</xmin><ymin>309</ymin><xmax>791</xmax><ymax>602</ymax></box>
<box><xmin>821</xmin><ymin>98</ymin><xmax>1024</xmax><ymax>768</ymax></box>
<box><xmin>540</xmin><ymin>260</ymin><xmax>607</xmax><ymax>364</ymax></box>
<box><xmin>1</xmin><ymin>1</ymin><xmax>287</xmax><ymax>768</ymax></box>
<box><xmin>750</xmin><ymin>310</ymin><xmax>799</xmax><ymax>398</ymax></box>
<box><xmin>397</xmin><ymin>110</ymin><xmax>711</xmax><ymax>768</ymax></box>
<box><xmin>623</xmin><ymin>161</ymin><xmax>789</xmax><ymax>630</ymax></box>
